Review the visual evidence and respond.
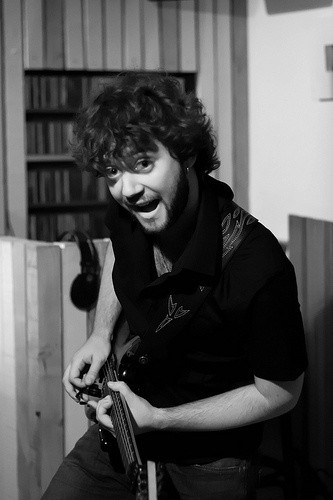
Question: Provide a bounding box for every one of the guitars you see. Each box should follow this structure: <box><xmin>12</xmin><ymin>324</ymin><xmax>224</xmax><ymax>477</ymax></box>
<box><xmin>73</xmin><ymin>304</ymin><xmax>163</xmax><ymax>500</ymax></box>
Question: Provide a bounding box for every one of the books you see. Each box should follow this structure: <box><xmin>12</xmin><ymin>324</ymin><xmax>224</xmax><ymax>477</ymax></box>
<box><xmin>26</xmin><ymin>75</ymin><xmax>197</xmax><ymax>245</ymax></box>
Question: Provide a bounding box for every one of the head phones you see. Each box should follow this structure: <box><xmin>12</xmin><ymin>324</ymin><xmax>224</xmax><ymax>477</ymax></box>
<box><xmin>56</xmin><ymin>229</ymin><xmax>102</xmax><ymax>311</ymax></box>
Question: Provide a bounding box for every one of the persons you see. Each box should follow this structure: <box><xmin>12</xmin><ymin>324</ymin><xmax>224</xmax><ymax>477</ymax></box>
<box><xmin>38</xmin><ymin>76</ymin><xmax>308</xmax><ymax>500</ymax></box>
